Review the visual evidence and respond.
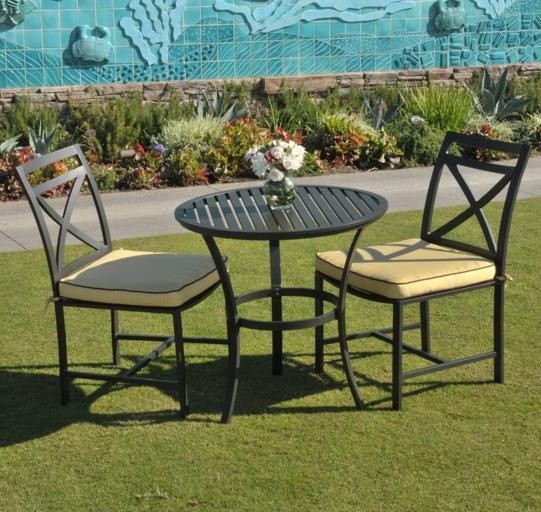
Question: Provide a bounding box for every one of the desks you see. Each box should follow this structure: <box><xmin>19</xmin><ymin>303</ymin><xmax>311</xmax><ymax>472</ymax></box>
<box><xmin>175</xmin><ymin>185</ymin><xmax>390</xmax><ymax>424</ymax></box>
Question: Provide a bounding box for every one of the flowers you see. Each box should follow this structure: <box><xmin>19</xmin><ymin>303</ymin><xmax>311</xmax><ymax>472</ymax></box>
<box><xmin>248</xmin><ymin>129</ymin><xmax>310</xmax><ymax>208</ymax></box>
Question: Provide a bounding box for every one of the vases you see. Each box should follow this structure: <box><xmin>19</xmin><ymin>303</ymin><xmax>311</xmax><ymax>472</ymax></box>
<box><xmin>264</xmin><ymin>178</ymin><xmax>297</xmax><ymax>210</ymax></box>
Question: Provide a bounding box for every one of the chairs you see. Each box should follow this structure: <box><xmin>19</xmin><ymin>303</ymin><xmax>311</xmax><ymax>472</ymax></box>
<box><xmin>313</xmin><ymin>129</ymin><xmax>533</xmax><ymax>412</ymax></box>
<box><xmin>12</xmin><ymin>141</ymin><xmax>230</xmax><ymax>421</ymax></box>
